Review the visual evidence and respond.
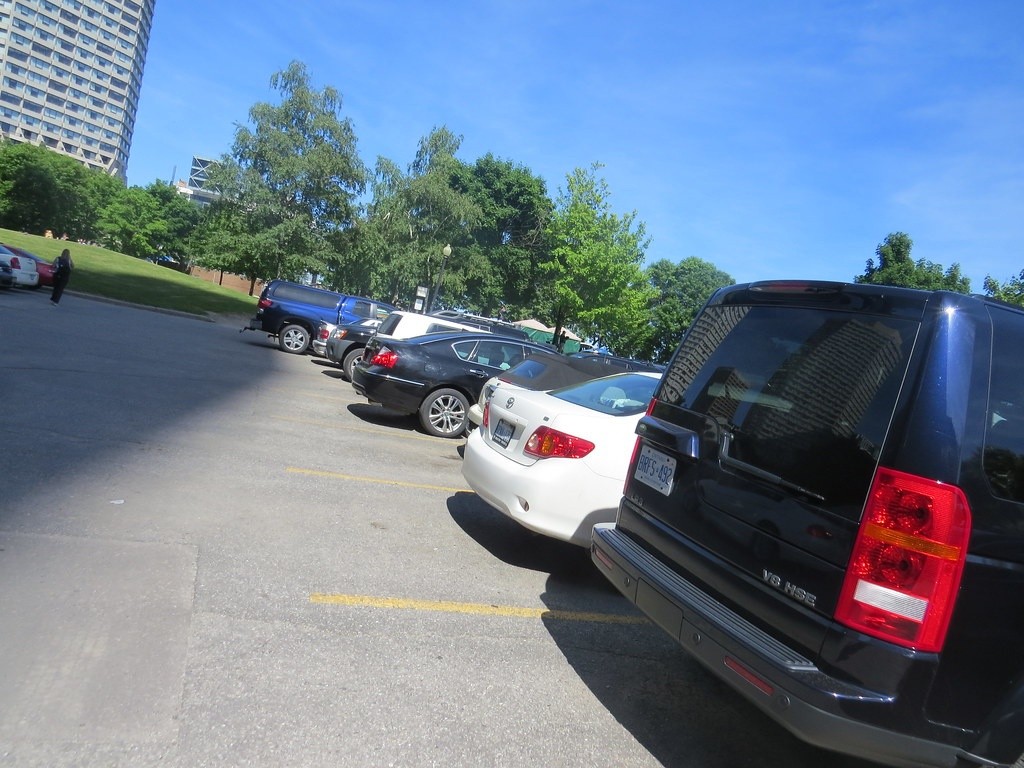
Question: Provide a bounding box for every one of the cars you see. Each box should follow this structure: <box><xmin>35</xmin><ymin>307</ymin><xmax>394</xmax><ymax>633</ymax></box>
<box><xmin>0</xmin><ymin>243</ymin><xmax>56</xmax><ymax>292</ymax></box>
<box><xmin>428</xmin><ymin>309</ymin><xmax>664</xmax><ymax>373</ymax></box>
<box><xmin>312</xmin><ymin>316</ymin><xmax>382</xmax><ymax>358</ymax></box>
<box><xmin>326</xmin><ymin>324</ymin><xmax>376</xmax><ymax>382</ymax></box>
<box><xmin>351</xmin><ymin>322</ymin><xmax>569</xmax><ymax>439</ymax></box>
<box><xmin>463</xmin><ymin>368</ymin><xmax>792</xmax><ymax>550</ymax></box>
<box><xmin>469</xmin><ymin>349</ymin><xmax>632</xmax><ymax>448</ymax></box>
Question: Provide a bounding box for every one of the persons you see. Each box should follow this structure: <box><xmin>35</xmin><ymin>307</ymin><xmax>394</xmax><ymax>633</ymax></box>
<box><xmin>49</xmin><ymin>249</ymin><xmax>74</xmax><ymax>306</ymax></box>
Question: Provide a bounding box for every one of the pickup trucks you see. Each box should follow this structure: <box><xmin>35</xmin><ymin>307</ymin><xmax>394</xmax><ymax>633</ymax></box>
<box><xmin>241</xmin><ymin>279</ymin><xmax>401</xmax><ymax>356</ymax></box>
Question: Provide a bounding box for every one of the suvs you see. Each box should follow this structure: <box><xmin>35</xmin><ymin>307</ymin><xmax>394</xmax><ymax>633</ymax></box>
<box><xmin>375</xmin><ymin>310</ymin><xmax>510</xmax><ymax>369</ymax></box>
<box><xmin>589</xmin><ymin>277</ymin><xmax>1023</xmax><ymax>768</ymax></box>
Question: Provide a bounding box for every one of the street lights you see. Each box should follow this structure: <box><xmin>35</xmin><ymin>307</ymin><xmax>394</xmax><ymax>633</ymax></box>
<box><xmin>431</xmin><ymin>243</ymin><xmax>452</xmax><ymax>309</ymax></box>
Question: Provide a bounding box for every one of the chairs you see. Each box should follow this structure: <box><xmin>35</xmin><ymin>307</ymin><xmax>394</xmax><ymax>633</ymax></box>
<box><xmin>593</xmin><ymin>385</ymin><xmax>625</xmax><ymax>406</ymax></box>
<box><xmin>507</xmin><ymin>354</ymin><xmax>521</xmax><ymax>371</ymax></box>
<box><xmin>494</xmin><ymin>351</ymin><xmax>505</xmax><ymax>367</ymax></box>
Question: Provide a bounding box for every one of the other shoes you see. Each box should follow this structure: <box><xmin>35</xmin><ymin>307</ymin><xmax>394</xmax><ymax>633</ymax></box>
<box><xmin>49</xmin><ymin>300</ymin><xmax>57</xmax><ymax>306</ymax></box>
